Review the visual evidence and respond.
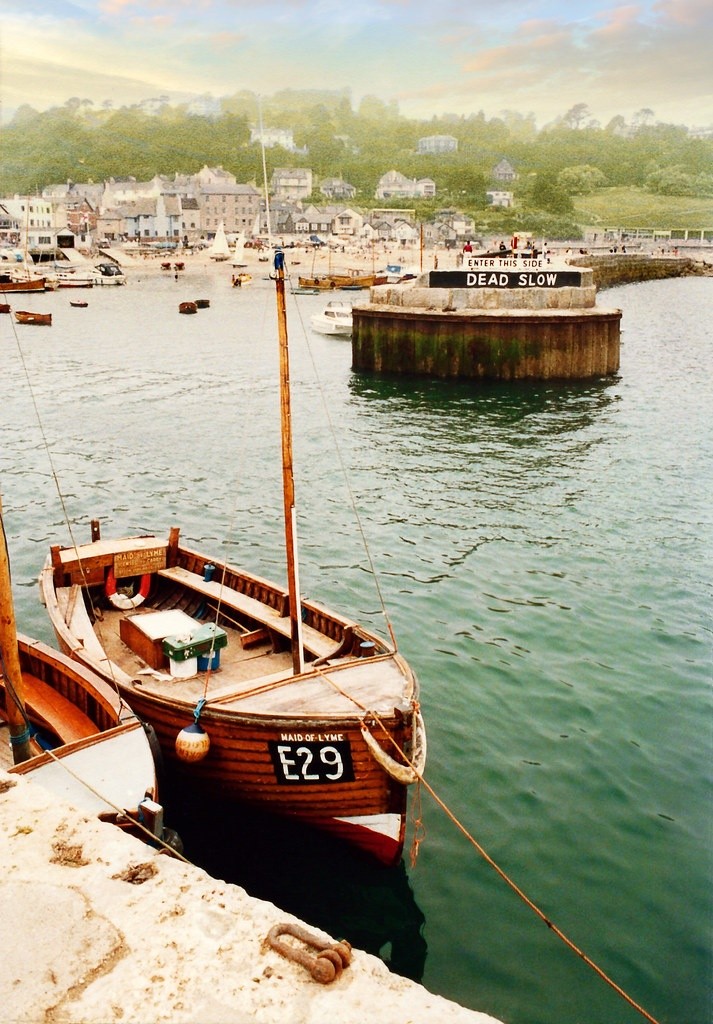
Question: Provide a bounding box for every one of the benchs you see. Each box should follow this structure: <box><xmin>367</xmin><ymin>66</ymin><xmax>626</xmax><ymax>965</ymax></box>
<box><xmin>157</xmin><ymin>565</ymin><xmax>358</xmax><ymax>666</ymax></box>
<box><xmin>0</xmin><ymin>661</ymin><xmax>100</xmax><ymax>743</ymax></box>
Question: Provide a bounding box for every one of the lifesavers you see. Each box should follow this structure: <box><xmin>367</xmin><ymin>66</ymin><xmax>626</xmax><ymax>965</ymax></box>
<box><xmin>104</xmin><ymin>565</ymin><xmax>151</xmax><ymax>610</ymax></box>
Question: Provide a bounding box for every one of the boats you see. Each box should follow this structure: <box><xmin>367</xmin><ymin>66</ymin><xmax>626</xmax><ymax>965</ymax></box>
<box><xmin>0</xmin><ymin>304</ymin><xmax>10</xmax><ymax>313</ymax></box>
<box><xmin>0</xmin><ymin>237</ymin><xmax>126</xmax><ymax>293</ymax></box>
<box><xmin>311</xmin><ymin>301</ymin><xmax>353</xmax><ymax>335</ymax></box>
<box><xmin>70</xmin><ymin>301</ymin><xmax>88</xmax><ymax>307</ymax></box>
<box><xmin>14</xmin><ymin>311</ymin><xmax>52</xmax><ymax>326</ymax></box>
<box><xmin>195</xmin><ymin>299</ymin><xmax>210</xmax><ymax>308</ymax></box>
<box><xmin>179</xmin><ymin>302</ymin><xmax>197</xmax><ymax>314</ymax></box>
<box><xmin>298</xmin><ymin>245</ymin><xmax>418</xmax><ymax>291</ymax></box>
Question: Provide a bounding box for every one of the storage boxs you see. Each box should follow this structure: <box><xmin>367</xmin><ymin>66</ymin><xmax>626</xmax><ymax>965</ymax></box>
<box><xmin>161</xmin><ymin>621</ymin><xmax>228</xmax><ymax>661</ymax></box>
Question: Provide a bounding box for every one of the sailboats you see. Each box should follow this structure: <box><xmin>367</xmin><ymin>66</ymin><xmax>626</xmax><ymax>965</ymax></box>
<box><xmin>207</xmin><ymin>220</ymin><xmax>232</xmax><ymax>261</ymax></box>
<box><xmin>223</xmin><ymin>228</ymin><xmax>248</xmax><ymax>266</ymax></box>
<box><xmin>38</xmin><ymin>250</ymin><xmax>426</xmax><ymax>868</ymax></box>
<box><xmin>0</xmin><ymin>289</ymin><xmax>164</xmax><ymax>840</ymax></box>
